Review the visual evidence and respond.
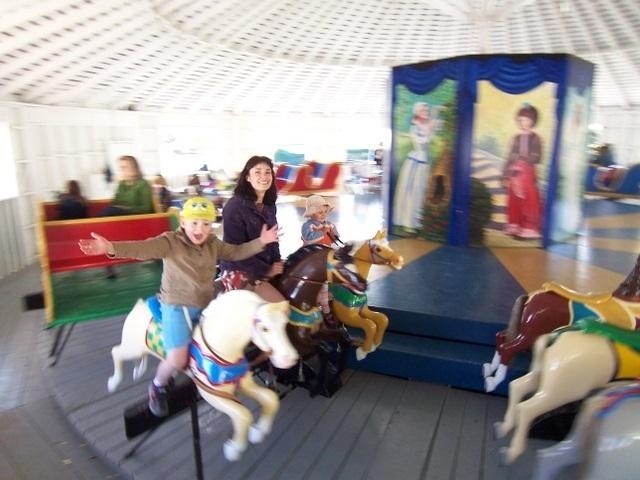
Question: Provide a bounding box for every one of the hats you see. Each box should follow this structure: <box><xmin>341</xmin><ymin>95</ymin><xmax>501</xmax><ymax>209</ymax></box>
<box><xmin>183</xmin><ymin>197</ymin><xmax>217</xmax><ymax>223</ymax></box>
<box><xmin>304</xmin><ymin>195</ymin><xmax>331</xmax><ymax>217</ymax></box>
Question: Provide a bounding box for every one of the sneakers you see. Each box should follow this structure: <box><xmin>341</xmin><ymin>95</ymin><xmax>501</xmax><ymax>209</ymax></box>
<box><xmin>147</xmin><ymin>378</ymin><xmax>172</xmax><ymax>419</ymax></box>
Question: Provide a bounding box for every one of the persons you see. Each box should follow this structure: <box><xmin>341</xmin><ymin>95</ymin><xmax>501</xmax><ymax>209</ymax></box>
<box><xmin>301</xmin><ymin>193</ymin><xmax>340</xmax><ymax>326</ymax></box>
<box><xmin>504</xmin><ymin>106</ymin><xmax>543</xmax><ymax>238</ymax></box>
<box><xmin>99</xmin><ymin>156</ymin><xmax>155</xmax><ymax>217</ymax></box>
<box><xmin>222</xmin><ymin>157</ymin><xmax>290</xmax><ymax>365</ymax></box>
<box><xmin>592</xmin><ymin>147</ymin><xmax>614</xmax><ymax>167</ymax></box>
<box><xmin>77</xmin><ymin>198</ymin><xmax>283</xmax><ymax>417</ymax></box>
<box><xmin>393</xmin><ymin>100</ymin><xmax>447</xmax><ymax>234</ymax></box>
<box><xmin>57</xmin><ymin>182</ymin><xmax>88</xmax><ymax>219</ymax></box>
